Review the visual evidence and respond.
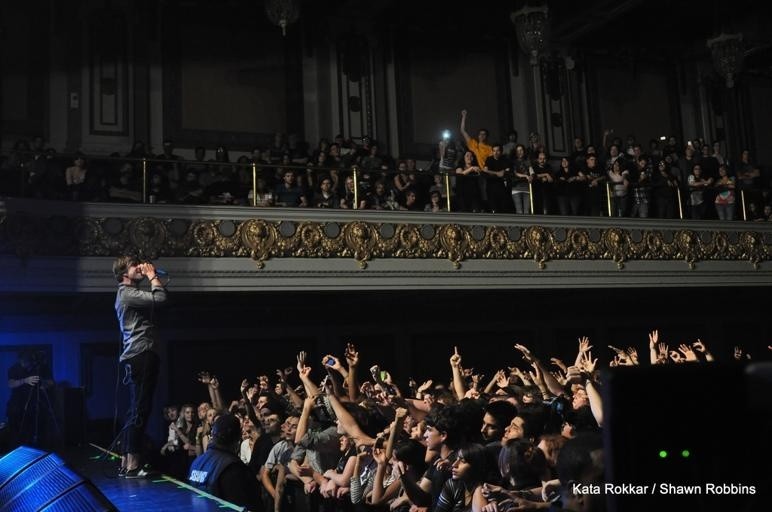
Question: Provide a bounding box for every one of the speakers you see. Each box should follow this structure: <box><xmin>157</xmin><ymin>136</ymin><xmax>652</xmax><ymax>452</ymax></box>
<box><xmin>0</xmin><ymin>444</ymin><xmax>118</xmax><ymax>511</ymax></box>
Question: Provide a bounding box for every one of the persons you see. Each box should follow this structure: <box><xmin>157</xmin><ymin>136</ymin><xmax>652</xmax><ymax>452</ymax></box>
<box><xmin>112</xmin><ymin>252</ymin><xmax>168</xmax><ymax>480</ymax></box>
<box><xmin>7</xmin><ymin>351</ymin><xmax>56</xmax><ymax>444</ymax></box>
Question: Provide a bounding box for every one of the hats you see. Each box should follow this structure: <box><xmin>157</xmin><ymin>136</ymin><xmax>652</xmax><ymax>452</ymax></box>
<box><xmin>211</xmin><ymin>415</ymin><xmax>241</xmax><ymax>444</ymax></box>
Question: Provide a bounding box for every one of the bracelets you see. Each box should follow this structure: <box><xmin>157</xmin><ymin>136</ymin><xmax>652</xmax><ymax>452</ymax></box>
<box><xmin>20</xmin><ymin>379</ymin><xmax>24</xmax><ymax>385</ymax></box>
<box><xmin>149</xmin><ymin>272</ymin><xmax>159</xmax><ymax>284</ymax></box>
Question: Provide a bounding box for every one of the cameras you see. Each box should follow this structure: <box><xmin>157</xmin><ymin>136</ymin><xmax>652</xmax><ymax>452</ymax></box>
<box><xmin>325</xmin><ymin>358</ymin><xmax>336</xmax><ymax>366</ymax></box>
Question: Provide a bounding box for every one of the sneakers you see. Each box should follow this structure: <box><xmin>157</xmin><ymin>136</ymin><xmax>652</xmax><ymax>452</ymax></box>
<box><xmin>118</xmin><ymin>465</ymin><xmax>160</xmax><ymax>479</ymax></box>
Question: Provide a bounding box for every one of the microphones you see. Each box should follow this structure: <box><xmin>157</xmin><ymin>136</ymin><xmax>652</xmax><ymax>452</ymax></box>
<box><xmin>154</xmin><ymin>270</ymin><xmax>167</xmax><ymax>277</ymax></box>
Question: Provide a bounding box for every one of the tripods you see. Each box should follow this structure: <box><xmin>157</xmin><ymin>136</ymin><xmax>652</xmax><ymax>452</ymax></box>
<box><xmin>17</xmin><ymin>383</ymin><xmax>60</xmax><ymax>443</ymax></box>
